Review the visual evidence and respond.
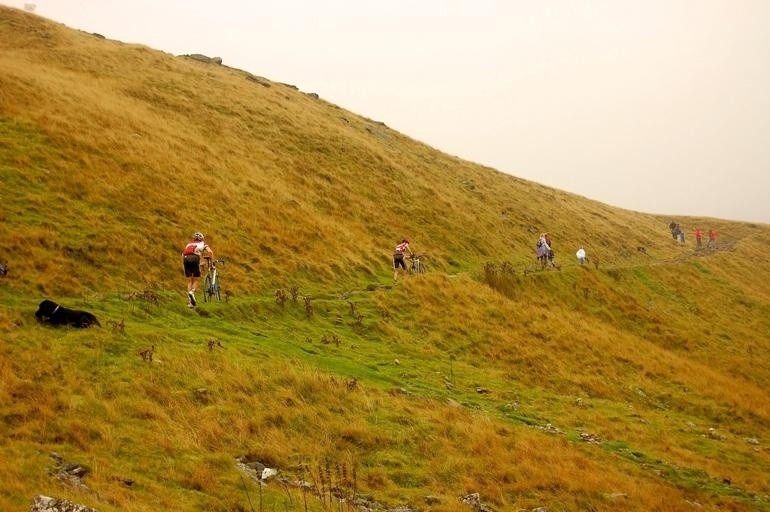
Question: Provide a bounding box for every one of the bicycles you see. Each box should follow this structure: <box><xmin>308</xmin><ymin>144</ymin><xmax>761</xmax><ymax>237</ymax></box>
<box><xmin>405</xmin><ymin>255</ymin><xmax>427</xmax><ymax>281</ymax></box>
<box><xmin>547</xmin><ymin>249</ymin><xmax>557</xmax><ymax>268</ymax></box>
<box><xmin>680</xmin><ymin>231</ymin><xmax>686</xmax><ymax>242</ymax></box>
<box><xmin>201</xmin><ymin>258</ymin><xmax>225</xmax><ymax>303</ymax></box>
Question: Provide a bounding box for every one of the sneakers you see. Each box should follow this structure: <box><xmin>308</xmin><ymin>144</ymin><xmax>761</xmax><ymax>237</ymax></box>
<box><xmin>187</xmin><ymin>291</ymin><xmax>197</xmax><ymax>308</ymax></box>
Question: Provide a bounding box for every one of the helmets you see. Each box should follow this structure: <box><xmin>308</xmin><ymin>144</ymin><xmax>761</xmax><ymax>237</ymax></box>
<box><xmin>193</xmin><ymin>232</ymin><xmax>204</xmax><ymax>241</ymax></box>
<box><xmin>402</xmin><ymin>239</ymin><xmax>409</xmax><ymax>244</ymax></box>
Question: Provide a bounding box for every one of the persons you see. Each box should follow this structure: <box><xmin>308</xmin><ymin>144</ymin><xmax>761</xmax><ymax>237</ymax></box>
<box><xmin>536</xmin><ymin>232</ymin><xmax>551</xmax><ymax>265</ymax></box>
<box><xmin>706</xmin><ymin>228</ymin><xmax>717</xmax><ymax>248</ymax></box>
<box><xmin>180</xmin><ymin>231</ymin><xmax>214</xmax><ymax>309</ymax></box>
<box><xmin>695</xmin><ymin>227</ymin><xmax>702</xmax><ymax>246</ymax></box>
<box><xmin>672</xmin><ymin>223</ymin><xmax>682</xmax><ymax>240</ymax></box>
<box><xmin>680</xmin><ymin>232</ymin><xmax>685</xmax><ymax>243</ymax></box>
<box><xmin>575</xmin><ymin>245</ymin><xmax>586</xmax><ymax>264</ymax></box>
<box><xmin>668</xmin><ymin>220</ymin><xmax>676</xmax><ymax>229</ymax></box>
<box><xmin>392</xmin><ymin>239</ymin><xmax>414</xmax><ymax>282</ymax></box>
<box><xmin>544</xmin><ymin>232</ymin><xmax>556</xmax><ymax>267</ymax></box>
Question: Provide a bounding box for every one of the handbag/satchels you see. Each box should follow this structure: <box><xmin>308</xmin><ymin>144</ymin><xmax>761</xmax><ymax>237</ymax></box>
<box><xmin>537</xmin><ymin>239</ymin><xmax>544</xmax><ymax>247</ymax></box>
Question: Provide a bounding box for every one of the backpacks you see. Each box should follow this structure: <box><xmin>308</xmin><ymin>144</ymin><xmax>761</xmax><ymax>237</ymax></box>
<box><xmin>183</xmin><ymin>245</ymin><xmax>197</xmax><ymax>255</ymax></box>
